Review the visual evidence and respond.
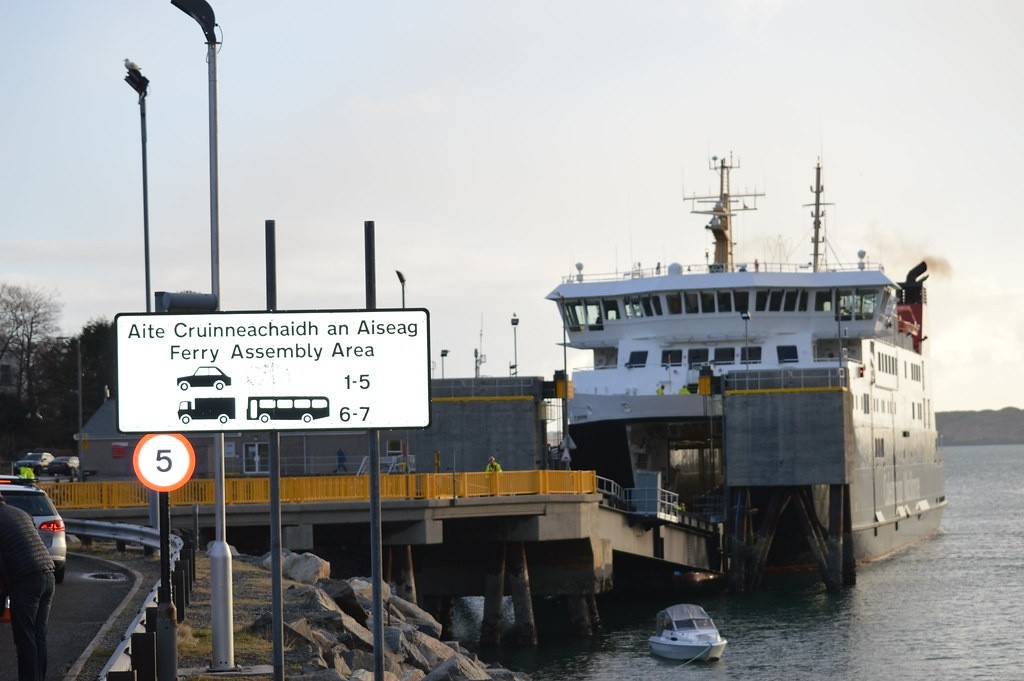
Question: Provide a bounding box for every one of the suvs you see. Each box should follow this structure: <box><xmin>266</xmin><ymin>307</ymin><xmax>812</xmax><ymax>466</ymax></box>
<box><xmin>13</xmin><ymin>452</ymin><xmax>57</xmax><ymax>477</ymax></box>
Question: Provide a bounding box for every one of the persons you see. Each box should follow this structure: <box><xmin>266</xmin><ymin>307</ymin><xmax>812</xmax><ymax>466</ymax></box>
<box><xmin>0</xmin><ymin>492</ymin><xmax>57</xmax><ymax>681</ymax></box>
<box><xmin>678</xmin><ymin>385</ymin><xmax>691</xmax><ymax>394</ymax></box>
<box><xmin>12</xmin><ymin>461</ymin><xmax>37</xmax><ymax>486</ymax></box>
<box><xmin>656</xmin><ymin>385</ymin><xmax>665</xmax><ymax>396</ymax></box>
<box><xmin>486</xmin><ymin>457</ymin><xmax>502</xmax><ymax>473</ymax></box>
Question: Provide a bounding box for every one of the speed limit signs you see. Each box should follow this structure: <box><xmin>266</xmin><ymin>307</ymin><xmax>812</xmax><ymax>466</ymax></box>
<box><xmin>134</xmin><ymin>434</ymin><xmax>197</xmax><ymax>493</ymax></box>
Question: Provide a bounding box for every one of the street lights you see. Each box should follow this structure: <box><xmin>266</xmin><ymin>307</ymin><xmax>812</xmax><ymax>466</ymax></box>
<box><xmin>57</xmin><ymin>336</ymin><xmax>85</xmax><ymax>483</ymax></box>
<box><xmin>509</xmin><ymin>312</ymin><xmax>521</xmax><ymax>377</ymax></box>
<box><xmin>440</xmin><ymin>350</ymin><xmax>450</xmax><ymax>378</ymax></box>
<box><xmin>122</xmin><ymin>57</ymin><xmax>155</xmax><ymax>317</ymax></box>
<box><xmin>171</xmin><ymin>0</ymin><xmax>238</xmax><ymax>678</ymax></box>
<box><xmin>396</xmin><ymin>269</ymin><xmax>406</xmax><ymax>311</ymax></box>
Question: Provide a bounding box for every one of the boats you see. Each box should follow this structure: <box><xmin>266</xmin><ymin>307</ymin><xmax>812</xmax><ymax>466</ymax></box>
<box><xmin>650</xmin><ymin>600</ymin><xmax>725</xmax><ymax>663</ymax></box>
<box><xmin>544</xmin><ymin>152</ymin><xmax>947</xmax><ymax>588</ymax></box>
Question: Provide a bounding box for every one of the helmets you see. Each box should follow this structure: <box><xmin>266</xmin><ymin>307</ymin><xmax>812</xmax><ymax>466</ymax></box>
<box><xmin>489</xmin><ymin>457</ymin><xmax>495</xmax><ymax>462</ymax></box>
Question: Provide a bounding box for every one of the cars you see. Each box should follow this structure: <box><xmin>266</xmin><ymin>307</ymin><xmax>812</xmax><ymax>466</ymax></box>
<box><xmin>46</xmin><ymin>456</ymin><xmax>81</xmax><ymax>477</ymax></box>
<box><xmin>0</xmin><ymin>472</ymin><xmax>67</xmax><ymax>583</ymax></box>
